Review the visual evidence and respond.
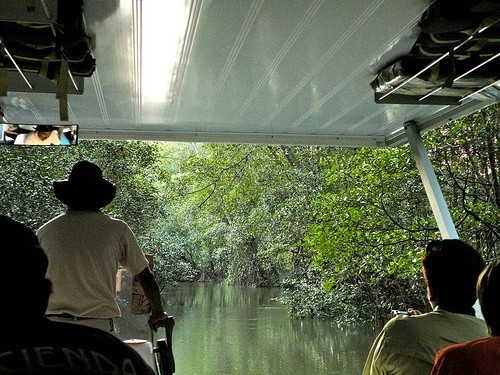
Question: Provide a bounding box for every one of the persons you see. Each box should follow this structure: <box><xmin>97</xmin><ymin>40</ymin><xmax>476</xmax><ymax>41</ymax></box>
<box><xmin>35</xmin><ymin>160</ymin><xmax>167</xmax><ymax>339</ymax></box>
<box><xmin>428</xmin><ymin>259</ymin><xmax>500</xmax><ymax>375</ymax></box>
<box><xmin>4</xmin><ymin>124</ymin><xmax>77</xmax><ymax>146</ymax></box>
<box><xmin>0</xmin><ymin>214</ymin><xmax>155</xmax><ymax>375</ymax></box>
<box><xmin>361</xmin><ymin>239</ymin><xmax>487</xmax><ymax>375</ymax></box>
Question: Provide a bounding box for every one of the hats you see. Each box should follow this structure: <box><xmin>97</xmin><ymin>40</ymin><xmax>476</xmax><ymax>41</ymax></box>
<box><xmin>52</xmin><ymin>161</ymin><xmax>117</xmax><ymax>210</ymax></box>
